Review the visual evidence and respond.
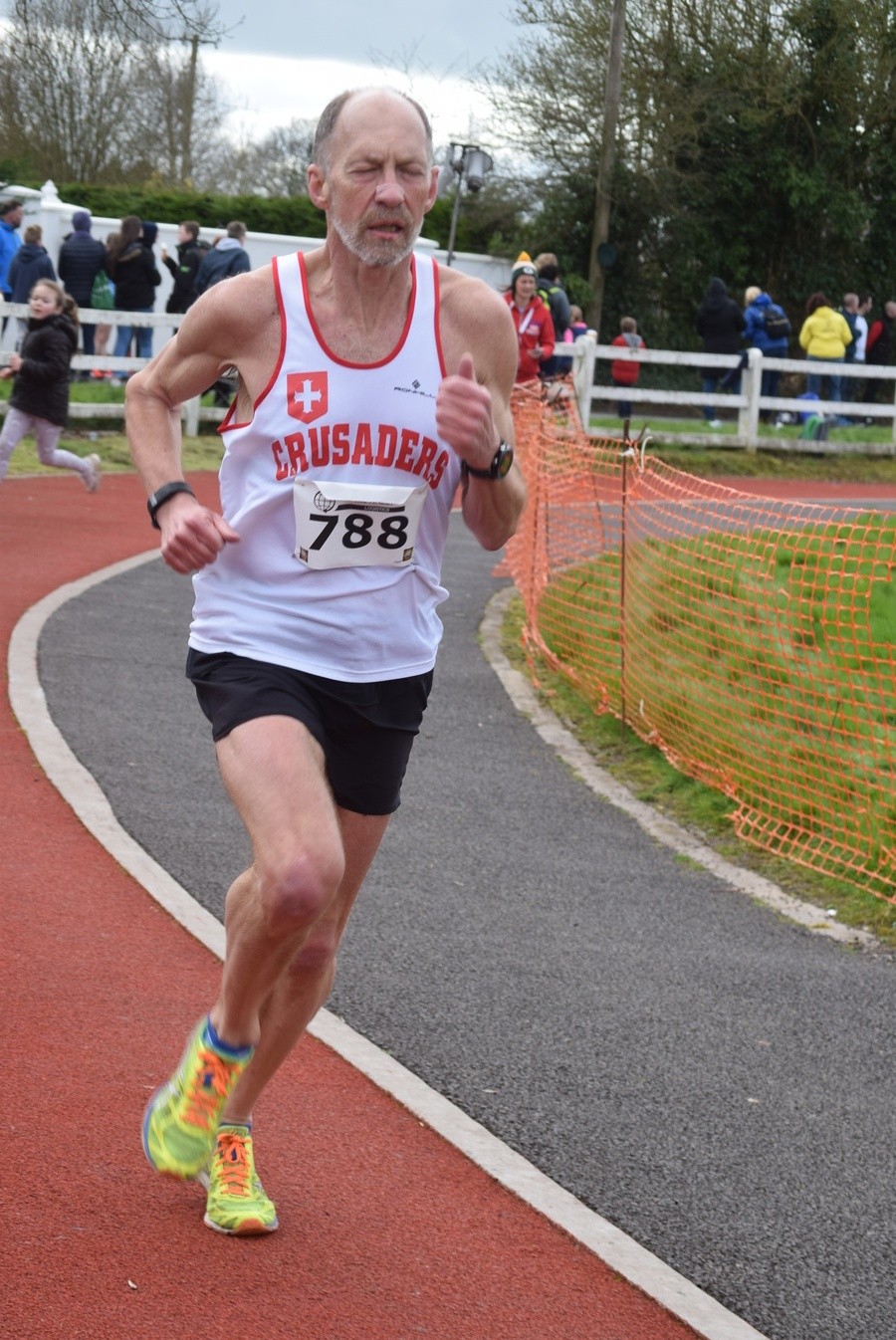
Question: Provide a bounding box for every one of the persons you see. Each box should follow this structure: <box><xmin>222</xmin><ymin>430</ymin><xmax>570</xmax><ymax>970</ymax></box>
<box><xmin>610</xmin><ymin>316</ymin><xmax>646</xmax><ymax>421</ymax></box>
<box><xmin>696</xmin><ymin>276</ymin><xmax>895</xmax><ymax>428</ymax></box>
<box><xmin>0</xmin><ymin>197</ymin><xmax>253</xmax><ymax>406</ymax></box>
<box><xmin>0</xmin><ymin>277</ymin><xmax>101</xmax><ymax>497</ymax></box>
<box><xmin>502</xmin><ymin>250</ymin><xmax>585</xmax><ymax>397</ymax></box>
<box><xmin>140</xmin><ymin>90</ymin><xmax>522</xmax><ymax>1233</ymax></box>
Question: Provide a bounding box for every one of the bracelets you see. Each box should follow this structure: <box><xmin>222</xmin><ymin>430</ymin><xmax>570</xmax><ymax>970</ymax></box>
<box><xmin>145</xmin><ymin>481</ymin><xmax>194</xmax><ymax>530</ymax></box>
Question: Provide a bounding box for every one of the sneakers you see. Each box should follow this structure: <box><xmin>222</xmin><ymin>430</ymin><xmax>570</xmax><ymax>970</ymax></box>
<box><xmin>142</xmin><ymin>1012</ymin><xmax>255</xmax><ymax>1181</ymax></box>
<box><xmin>81</xmin><ymin>453</ymin><xmax>102</xmax><ymax>494</ymax></box>
<box><xmin>195</xmin><ymin>1122</ymin><xmax>279</xmax><ymax>1234</ymax></box>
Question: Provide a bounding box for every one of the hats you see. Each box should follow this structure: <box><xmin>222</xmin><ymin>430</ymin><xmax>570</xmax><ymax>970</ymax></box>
<box><xmin>512</xmin><ymin>251</ymin><xmax>538</xmax><ymax>281</ymax></box>
<box><xmin>73</xmin><ymin>211</ymin><xmax>91</xmax><ymax>233</ymax></box>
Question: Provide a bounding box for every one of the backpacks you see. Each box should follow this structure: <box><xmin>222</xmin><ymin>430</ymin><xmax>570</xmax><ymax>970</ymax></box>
<box><xmin>89</xmin><ymin>270</ymin><xmax>116</xmax><ymax>309</ymax></box>
<box><xmin>754</xmin><ymin>304</ymin><xmax>791</xmax><ymax>339</ymax></box>
<box><xmin>797</xmin><ymin>414</ymin><xmax>828</xmax><ymax>460</ymax></box>
<box><xmin>536</xmin><ymin>287</ymin><xmax>562</xmax><ymax>312</ymax></box>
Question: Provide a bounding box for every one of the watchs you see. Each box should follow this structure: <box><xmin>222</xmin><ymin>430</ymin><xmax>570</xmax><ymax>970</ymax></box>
<box><xmin>464</xmin><ymin>440</ymin><xmax>513</xmax><ymax>479</ymax></box>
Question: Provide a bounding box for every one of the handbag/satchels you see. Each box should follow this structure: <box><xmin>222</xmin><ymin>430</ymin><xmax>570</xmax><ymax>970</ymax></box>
<box><xmin>715</xmin><ymin>325</ymin><xmax>740</xmax><ymax>351</ymax></box>
